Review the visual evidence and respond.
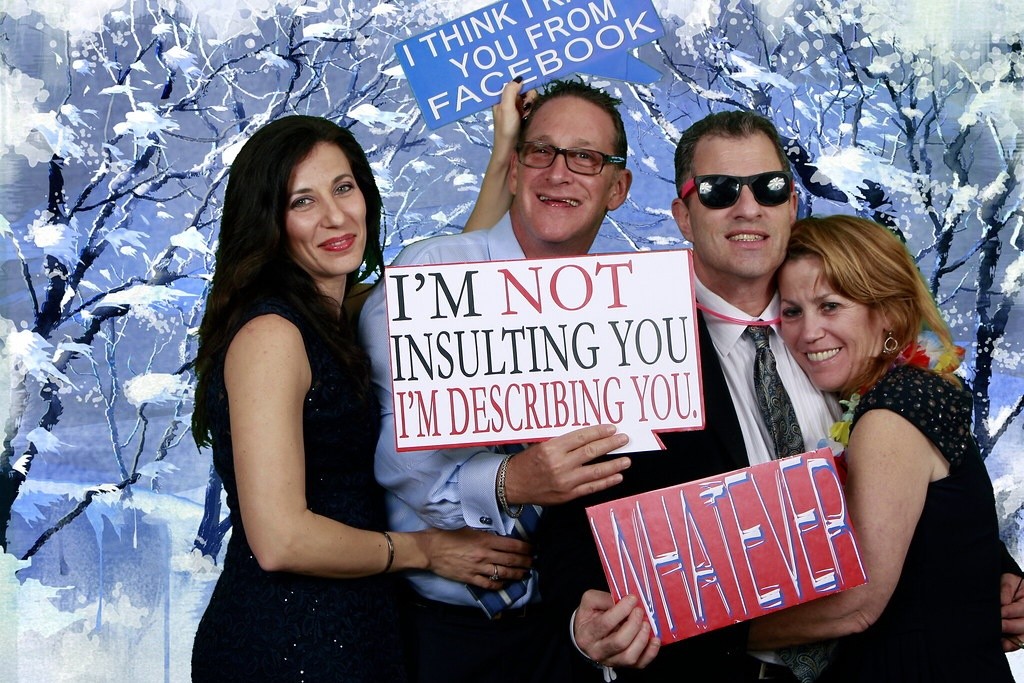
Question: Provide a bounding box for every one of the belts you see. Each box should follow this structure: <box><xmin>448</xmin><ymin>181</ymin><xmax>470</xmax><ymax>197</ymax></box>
<box><xmin>744</xmin><ymin>654</ymin><xmax>793</xmax><ymax>680</ymax></box>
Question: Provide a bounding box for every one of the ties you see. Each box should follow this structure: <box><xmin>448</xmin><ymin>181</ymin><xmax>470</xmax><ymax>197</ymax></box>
<box><xmin>746</xmin><ymin>325</ymin><xmax>805</xmax><ymax>459</ymax></box>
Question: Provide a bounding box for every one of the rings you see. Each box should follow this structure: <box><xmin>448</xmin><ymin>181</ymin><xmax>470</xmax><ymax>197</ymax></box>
<box><xmin>489</xmin><ymin>564</ymin><xmax>499</xmax><ymax>582</ymax></box>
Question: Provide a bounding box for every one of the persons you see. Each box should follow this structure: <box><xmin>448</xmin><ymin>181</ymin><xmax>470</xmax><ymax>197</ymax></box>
<box><xmin>191</xmin><ymin>77</ymin><xmax>1024</xmax><ymax>683</ymax></box>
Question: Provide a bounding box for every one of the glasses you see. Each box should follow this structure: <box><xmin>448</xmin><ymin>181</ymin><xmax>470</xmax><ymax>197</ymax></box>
<box><xmin>681</xmin><ymin>170</ymin><xmax>793</xmax><ymax>209</ymax></box>
<box><xmin>516</xmin><ymin>141</ymin><xmax>626</xmax><ymax>176</ymax></box>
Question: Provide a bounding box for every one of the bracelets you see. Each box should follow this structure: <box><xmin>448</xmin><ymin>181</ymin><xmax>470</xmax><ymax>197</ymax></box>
<box><xmin>379</xmin><ymin>532</ymin><xmax>394</xmax><ymax>575</ymax></box>
<box><xmin>498</xmin><ymin>452</ymin><xmax>524</xmax><ymax>518</ymax></box>
